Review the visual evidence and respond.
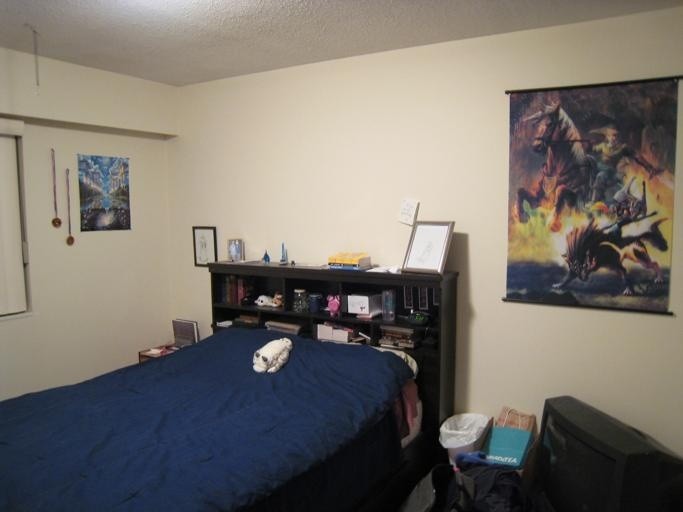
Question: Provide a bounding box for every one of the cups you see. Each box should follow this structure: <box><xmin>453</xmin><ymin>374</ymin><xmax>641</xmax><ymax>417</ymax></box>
<box><xmin>381</xmin><ymin>289</ymin><xmax>395</xmax><ymax>321</ymax></box>
<box><xmin>308</xmin><ymin>294</ymin><xmax>322</xmax><ymax>314</ymax></box>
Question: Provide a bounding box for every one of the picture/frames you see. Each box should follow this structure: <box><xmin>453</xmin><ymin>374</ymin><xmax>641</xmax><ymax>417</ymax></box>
<box><xmin>227</xmin><ymin>238</ymin><xmax>244</xmax><ymax>263</ymax></box>
<box><xmin>192</xmin><ymin>226</ymin><xmax>218</xmax><ymax>267</ymax></box>
<box><xmin>401</xmin><ymin>220</ymin><xmax>455</xmax><ymax>276</ymax></box>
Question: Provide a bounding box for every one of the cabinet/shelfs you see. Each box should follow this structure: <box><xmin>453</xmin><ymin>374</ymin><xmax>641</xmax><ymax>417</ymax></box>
<box><xmin>206</xmin><ymin>260</ymin><xmax>460</xmax><ymax>436</ymax></box>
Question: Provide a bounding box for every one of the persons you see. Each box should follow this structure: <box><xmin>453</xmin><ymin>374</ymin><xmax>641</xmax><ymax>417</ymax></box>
<box><xmin>228</xmin><ymin>240</ymin><xmax>235</xmax><ymax>260</ymax></box>
<box><xmin>585</xmin><ymin>122</ymin><xmax>664</xmax><ymax>207</ymax></box>
<box><xmin>234</xmin><ymin>239</ymin><xmax>241</xmax><ymax>260</ymax></box>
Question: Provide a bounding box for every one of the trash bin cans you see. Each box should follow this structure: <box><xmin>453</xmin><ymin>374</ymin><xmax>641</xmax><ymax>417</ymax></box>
<box><xmin>438</xmin><ymin>412</ymin><xmax>495</xmax><ymax>468</ymax></box>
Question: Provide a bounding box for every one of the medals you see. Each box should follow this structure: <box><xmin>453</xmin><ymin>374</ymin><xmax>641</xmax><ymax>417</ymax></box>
<box><xmin>51</xmin><ymin>218</ymin><xmax>61</xmax><ymax>227</ymax></box>
<box><xmin>64</xmin><ymin>236</ymin><xmax>74</xmax><ymax>246</ymax></box>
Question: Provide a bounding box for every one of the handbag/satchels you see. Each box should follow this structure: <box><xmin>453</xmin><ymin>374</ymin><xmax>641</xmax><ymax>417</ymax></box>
<box><xmin>486</xmin><ymin>409</ymin><xmax>534</xmax><ymax>467</ymax></box>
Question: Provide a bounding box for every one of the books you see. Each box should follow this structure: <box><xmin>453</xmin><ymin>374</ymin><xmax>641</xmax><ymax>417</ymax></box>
<box><xmin>328</xmin><ymin>265</ymin><xmax>372</xmax><ymax>271</ymax></box>
<box><xmin>171</xmin><ymin>318</ymin><xmax>200</xmax><ymax>347</ymax></box>
<box><xmin>215</xmin><ymin>272</ymin><xmax>421</xmax><ymax>351</ymax></box>
<box><xmin>328</xmin><ymin>252</ymin><xmax>371</xmax><ymax>265</ymax></box>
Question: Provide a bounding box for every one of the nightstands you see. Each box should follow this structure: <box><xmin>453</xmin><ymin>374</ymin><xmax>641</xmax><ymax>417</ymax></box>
<box><xmin>136</xmin><ymin>343</ymin><xmax>183</xmax><ymax>366</ymax></box>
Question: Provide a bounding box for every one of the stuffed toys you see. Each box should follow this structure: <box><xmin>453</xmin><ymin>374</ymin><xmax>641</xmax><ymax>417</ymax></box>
<box><xmin>250</xmin><ymin>336</ymin><xmax>294</xmax><ymax>374</ymax></box>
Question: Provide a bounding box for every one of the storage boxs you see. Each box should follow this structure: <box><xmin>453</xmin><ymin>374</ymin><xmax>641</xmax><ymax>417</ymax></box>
<box><xmin>315</xmin><ymin>323</ymin><xmax>332</xmax><ymax>341</ymax></box>
<box><xmin>347</xmin><ymin>293</ymin><xmax>382</xmax><ymax>315</ymax></box>
<box><xmin>331</xmin><ymin>327</ymin><xmax>352</xmax><ymax>343</ymax></box>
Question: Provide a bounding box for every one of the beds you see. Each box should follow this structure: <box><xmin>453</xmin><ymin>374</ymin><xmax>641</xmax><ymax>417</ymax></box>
<box><xmin>2</xmin><ymin>326</ymin><xmax>427</xmax><ymax>512</ymax></box>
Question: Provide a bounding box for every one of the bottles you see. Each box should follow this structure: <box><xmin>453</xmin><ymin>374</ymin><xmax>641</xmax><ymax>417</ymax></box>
<box><xmin>293</xmin><ymin>289</ymin><xmax>307</xmax><ymax>313</ymax></box>
